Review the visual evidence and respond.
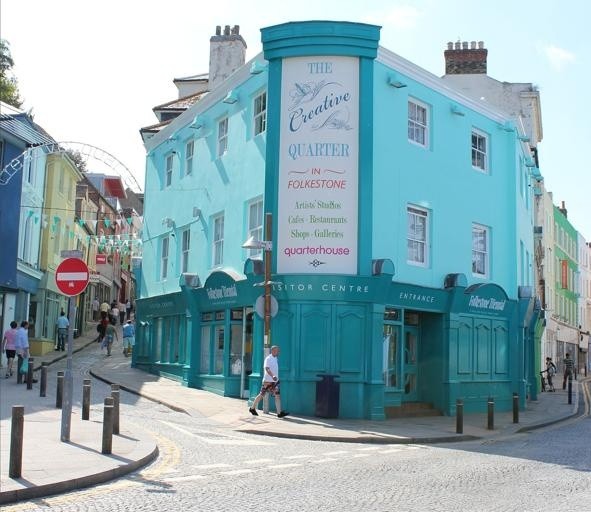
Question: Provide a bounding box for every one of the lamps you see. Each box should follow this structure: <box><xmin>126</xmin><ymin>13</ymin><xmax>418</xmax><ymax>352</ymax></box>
<box><xmin>250</xmin><ymin>61</ymin><xmax>264</xmax><ymax>74</ymax></box>
<box><xmin>166</xmin><ymin>134</ymin><xmax>176</xmax><ymax>145</ymax></box>
<box><xmin>450</xmin><ymin>102</ymin><xmax>465</xmax><ymax>116</ymax></box>
<box><xmin>222</xmin><ymin>90</ymin><xmax>239</xmax><ymax>104</ymax></box>
<box><xmin>504</xmin><ymin>120</ymin><xmax>544</xmax><ymax>197</ymax></box>
<box><xmin>189</xmin><ymin>115</ymin><xmax>203</xmax><ymax>129</ymax></box>
<box><xmin>193</xmin><ymin>207</ymin><xmax>201</xmax><ymax>217</ymax></box>
<box><xmin>389</xmin><ymin>73</ymin><xmax>406</xmax><ymax>89</ymax></box>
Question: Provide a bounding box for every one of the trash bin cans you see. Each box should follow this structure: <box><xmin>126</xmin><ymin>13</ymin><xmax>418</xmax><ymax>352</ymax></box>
<box><xmin>315</xmin><ymin>374</ymin><xmax>340</xmax><ymax>418</ymax></box>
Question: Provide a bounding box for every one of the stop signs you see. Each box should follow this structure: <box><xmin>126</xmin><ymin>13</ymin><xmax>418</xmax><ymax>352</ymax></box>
<box><xmin>54</xmin><ymin>256</ymin><xmax>90</xmax><ymax>296</ymax></box>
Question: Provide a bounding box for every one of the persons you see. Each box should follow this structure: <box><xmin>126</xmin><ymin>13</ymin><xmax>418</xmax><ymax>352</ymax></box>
<box><xmin>561</xmin><ymin>352</ymin><xmax>575</xmax><ymax>391</ymax></box>
<box><xmin>97</xmin><ymin>315</ymin><xmax>109</xmax><ymax>342</ymax></box>
<box><xmin>104</xmin><ymin>318</ymin><xmax>119</xmax><ymax>357</ymax></box>
<box><xmin>545</xmin><ymin>358</ymin><xmax>557</xmax><ymax>392</ymax></box>
<box><xmin>55</xmin><ymin>311</ymin><xmax>69</xmax><ymax>351</ymax></box>
<box><xmin>122</xmin><ymin>319</ymin><xmax>136</xmax><ymax>357</ymax></box>
<box><xmin>1</xmin><ymin>321</ymin><xmax>18</xmax><ymax>379</ymax></box>
<box><xmin>14</xmin><ymin>319</ymin><xmax>39</xmax><ymax>383</ymax></box>
<box><xmin>248</xmin><ymin>344</ymin><xmax>290</xmax><ymax>419</ymax></box>
<box><xmin>92</xmin><ymin>296</ymin><xmax>137</xmax><ymax>324</ymax></box>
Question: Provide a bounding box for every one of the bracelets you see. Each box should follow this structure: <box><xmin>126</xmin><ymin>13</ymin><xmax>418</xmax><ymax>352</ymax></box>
<box><xmin>273</xmin><ymin>376</ymin><xmax>275</xmax><ymax>378</ymax></box>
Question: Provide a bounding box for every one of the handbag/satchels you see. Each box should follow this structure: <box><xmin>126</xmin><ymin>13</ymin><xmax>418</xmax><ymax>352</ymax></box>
<box><xmin>20</xmin><ymin>358</ymin><xmax>28</xmax><ymax>374</ymax></box>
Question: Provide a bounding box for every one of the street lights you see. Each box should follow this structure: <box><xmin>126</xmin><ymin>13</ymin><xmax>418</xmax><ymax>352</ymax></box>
<box><xmin>239</xmin><ymin>233</ymin><xmax>272</xmax><ymax>415</ymax></box>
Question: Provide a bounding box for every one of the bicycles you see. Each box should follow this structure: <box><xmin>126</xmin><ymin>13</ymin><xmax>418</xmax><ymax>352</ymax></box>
<box><xmin>540</xmin><ymin>370</ymin><xmax>550</xmax><ymax>393</ymax></box>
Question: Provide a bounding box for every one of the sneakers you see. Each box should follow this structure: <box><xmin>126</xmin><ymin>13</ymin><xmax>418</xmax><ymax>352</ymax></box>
<box><xmin>249</xmin><ymin>407</ymin><xmax>258</xmax><ymax>415</ymax></box>
<box><xmin>278</xmin><ymin>412</ymin><xmax>289</xmax><ymax>417</ymax></box>
